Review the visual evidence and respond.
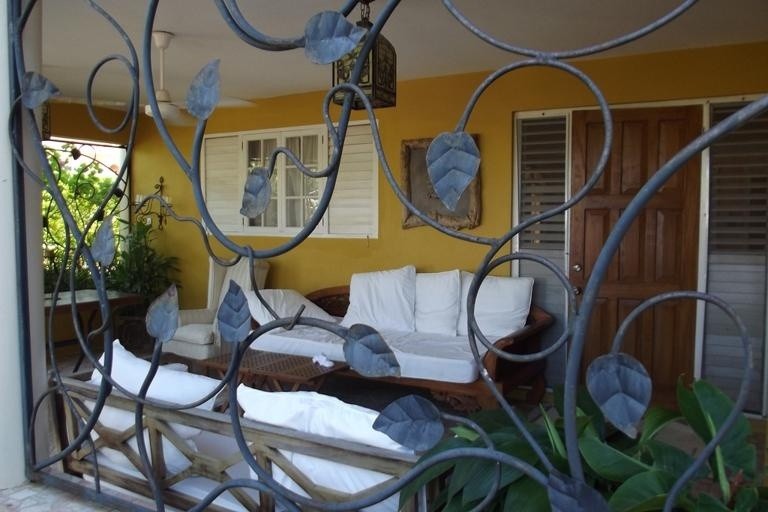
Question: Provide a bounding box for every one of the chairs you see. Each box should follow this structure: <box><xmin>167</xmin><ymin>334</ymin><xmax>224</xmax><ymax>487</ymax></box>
<box><xmin>151</xmin><ymin>250</ymin><xmax>272</xmax><ymax>372</ymax></box>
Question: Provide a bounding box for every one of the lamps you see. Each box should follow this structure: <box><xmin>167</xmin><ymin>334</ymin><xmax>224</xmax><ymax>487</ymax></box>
<box><xmin>130</xmin><ymin>175</ymin><xmax>174</xmax><ymax>231</ymax></box>
<box><xmin>329</xmin><ymin>0</ymin><xmax>395</xmax><ymax>114</ymax></box>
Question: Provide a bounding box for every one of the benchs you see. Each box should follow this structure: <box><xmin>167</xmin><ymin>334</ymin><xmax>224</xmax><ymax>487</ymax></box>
<box><xmin>51</xmin><ymin>353</ymin><xmax>452</xmax><ymax>512</ymax></box>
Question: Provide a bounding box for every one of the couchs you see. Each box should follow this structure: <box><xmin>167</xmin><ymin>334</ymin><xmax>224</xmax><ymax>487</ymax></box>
<box><xmin>244</xmin><ymin>268</ymin><xmax>559</xmax><ymax>421</ymax></box>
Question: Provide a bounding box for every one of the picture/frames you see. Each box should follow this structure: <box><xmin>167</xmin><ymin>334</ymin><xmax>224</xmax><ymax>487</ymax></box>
<box><xmin>399</xmin><ymin>132</ymin><xmax>482</xmax><ymax>230</ymax></box>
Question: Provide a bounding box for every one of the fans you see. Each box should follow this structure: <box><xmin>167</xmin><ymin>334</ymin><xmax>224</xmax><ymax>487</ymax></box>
<box><xmin>46</xmin><ymin>27</ymin><xmax>260</xmax><ymax>130</ymax></box>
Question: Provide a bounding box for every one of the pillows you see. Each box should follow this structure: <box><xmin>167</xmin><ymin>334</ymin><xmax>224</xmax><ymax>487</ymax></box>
<box><xmin>409</xmin><ymin>266</ymin><xmax>461</xmax><ymax>339</ymax></box>
<box><xmin>233</xmin><ymin>380</ymin><xmax>417</xmax><ymax>511</ymax></box>
<box><xmin>78</xmin><ymin>337</ymin><xmax>231</xmax><ymax>475</ymax></box>
<box><xmin>338</xmin><ymin>262</ymin><xmax>418</xmax><ymax>336</ymax></box>
<box><xmin>457</xmin><ymin>270</ymin><xmax>535</xmax><ymax>339</ymax></box>
<box><xmin>242</xmin><ymin>286</ymin><xmax>338</xmax><ymax>332</ymax></box>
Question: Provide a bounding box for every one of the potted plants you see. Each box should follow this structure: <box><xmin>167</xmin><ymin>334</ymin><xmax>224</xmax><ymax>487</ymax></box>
<box><xmin>95</xmin><ymin>218</ymin><xmax>184</xmax><ymax>348</ymax></box>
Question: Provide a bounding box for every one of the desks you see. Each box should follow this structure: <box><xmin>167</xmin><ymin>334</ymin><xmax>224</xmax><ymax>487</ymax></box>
<box><xmin>45</xmin><ymin>288</ymin><xmax>148</xmax><ymax>375</ymax></box>
<box><xmin>194</xmin><ymin>344</ymin><xmax>352</xmax><ymax>397</ymax></box>
